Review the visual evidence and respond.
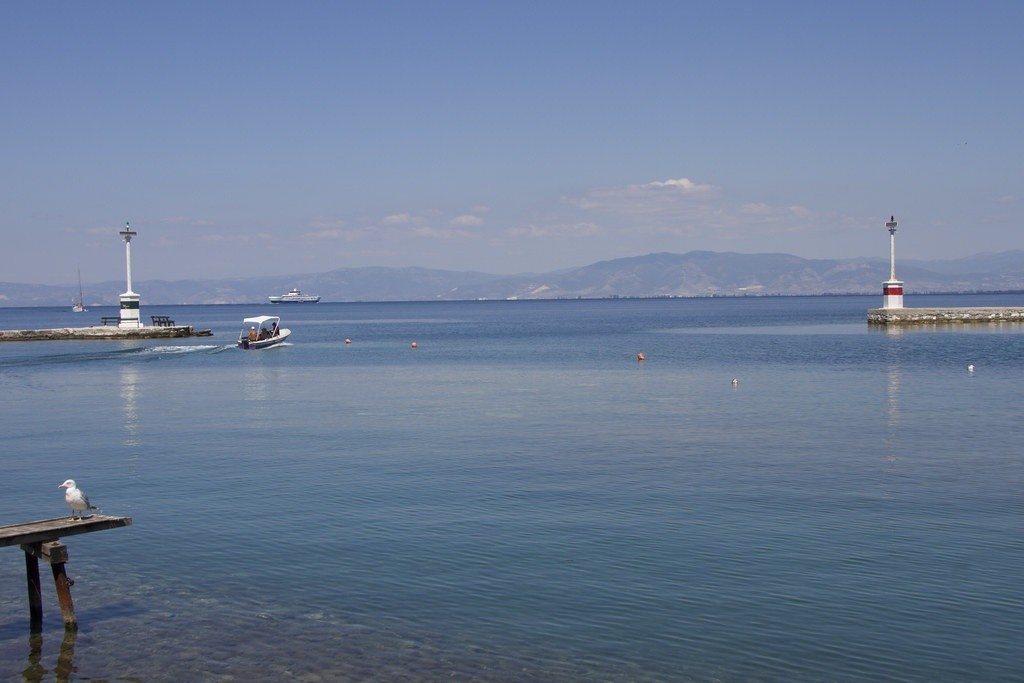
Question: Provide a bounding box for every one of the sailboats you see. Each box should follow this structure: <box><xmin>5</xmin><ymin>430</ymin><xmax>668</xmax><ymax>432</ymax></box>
<box><xmin>71</xmin><ymin>270</ymin><xmax>89</xmax><ymax>313</ymax></box>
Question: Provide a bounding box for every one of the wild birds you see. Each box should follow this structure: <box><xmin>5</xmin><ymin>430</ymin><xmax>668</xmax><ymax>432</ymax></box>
<box><xmin>58</xmin><ymin>479</ymin><xmax>99</xmax><ymax>520</ymax></box>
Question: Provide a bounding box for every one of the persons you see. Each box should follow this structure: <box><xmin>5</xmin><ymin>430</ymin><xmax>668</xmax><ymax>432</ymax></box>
<box><xmin>272</xmin><ymin>322</ymin><xmax>279</xmax><ymax>337</ymax></box>
<box><xmin>259</xmin><ymin>327</ymin><xmax>272</xmax><ymax>340</ymax></box>
<box><xmin>248</xmin><ymin>326</ymin><xmax>258</xmax><ymax>341</ymax></box>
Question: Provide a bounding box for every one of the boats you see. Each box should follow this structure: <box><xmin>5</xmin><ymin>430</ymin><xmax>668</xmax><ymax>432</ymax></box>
<box><xmin>268</xmin><ymin>288</ymin><xmax>322</xmax><ymax>304</ymax></box>
<box><xmin>237</xmin><ymin>316</ymin><xmax>292</xmax><ymax>351</ymax></box>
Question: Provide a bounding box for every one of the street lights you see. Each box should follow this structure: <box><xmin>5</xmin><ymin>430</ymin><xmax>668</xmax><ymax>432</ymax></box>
<box><xmin>116</xmin><ymin>221</ymin><xmax>144</xmax><ymax>329</ymax></box>
<box><xmin>882</xmin><ymin>215</ymin><xmax>904</xmax><ymax>307</ymax></box>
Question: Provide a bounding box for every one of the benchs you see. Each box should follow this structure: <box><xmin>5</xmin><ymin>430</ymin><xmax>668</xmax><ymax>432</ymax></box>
<box><xmin>101</xmin><ymin>317</ymin><xmax>121</xmax><ymax>326</ymax></box>
<box><xmin>151</xmin><ymin>316</ymin><xmax>175</xmax><ymax>327</ymax></box>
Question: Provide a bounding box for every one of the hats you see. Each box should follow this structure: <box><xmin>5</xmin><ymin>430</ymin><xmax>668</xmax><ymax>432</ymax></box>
<box><xmin>249</xmin><ymin>327</ymin><xmax>255</xmax><ymax>330</ymax></box>
<box><xmin>261</xmin><ymin>328</ymin><xmax>266</xmax><ymax>330</ymax></box>
<box><xmin>272</xmin><ymin>322</ymin><xmax>276</xmax><ymax>325</ymax></box>
